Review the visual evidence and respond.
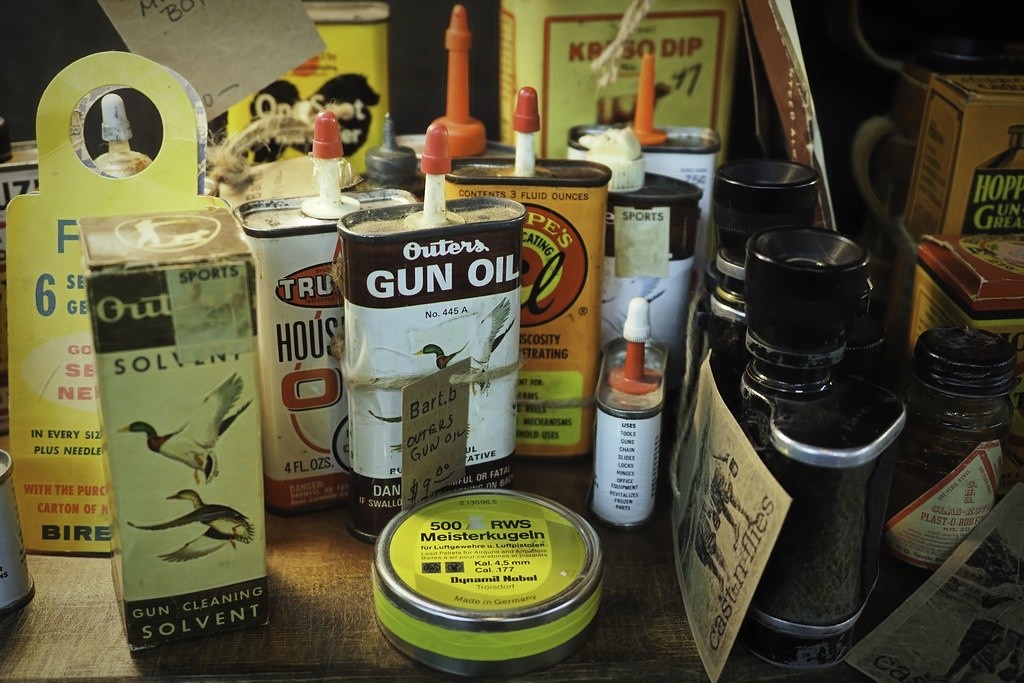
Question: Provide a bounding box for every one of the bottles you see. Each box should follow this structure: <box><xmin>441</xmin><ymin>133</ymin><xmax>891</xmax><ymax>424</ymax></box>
<box><xmin>220</xmin><ymin>0</ymin><xmax>1014</xmax><ymax>671</ymax></box>
